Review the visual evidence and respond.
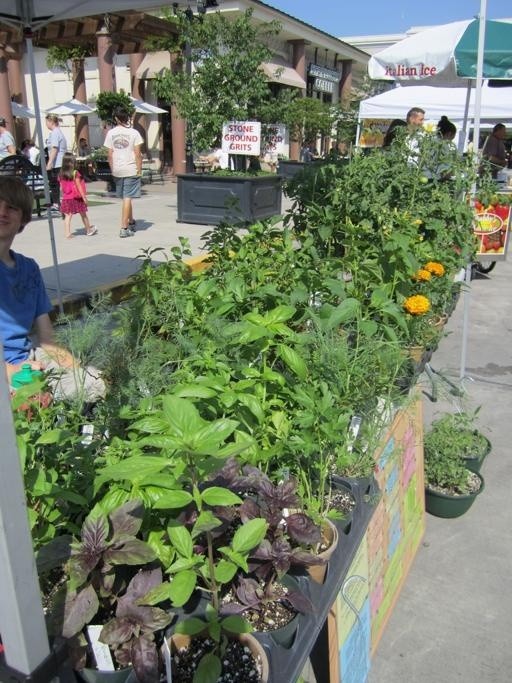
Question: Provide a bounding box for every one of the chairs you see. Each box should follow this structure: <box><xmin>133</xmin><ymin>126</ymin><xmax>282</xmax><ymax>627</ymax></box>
<box><xmin>0</xmin><ymin>149</ymin><xmax>167</xmax><ymax>219</ymax></box>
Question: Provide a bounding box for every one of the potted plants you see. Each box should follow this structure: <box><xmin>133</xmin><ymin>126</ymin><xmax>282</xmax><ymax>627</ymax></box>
<box><xmin>425</xmin><ymin>453</ymin><xmax>484</xmax><ymax>518</ymax></box>
<box><xmin>151</xmin><ymin>0</ymin><xmax>297</xmax><ymax>230</ymax></box>
<box><xmin>278</xmin><ymin>96</ymin><xmax>354</xmax><ymax>183</ymax></box>
<box><xmin>424</xmin><ymin>406</ymin><xmax>491</xmax><ymax>472</ymax></box>
<box><xmin>10</xmin><ymin>221</ymin><xmax>412</xmax><ymax>683</ymax></box>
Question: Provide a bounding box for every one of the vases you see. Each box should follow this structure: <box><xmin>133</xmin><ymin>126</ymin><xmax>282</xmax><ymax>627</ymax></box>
<box><xmin>398</xmin><ymin>337</ymin><xmax>425</xmax><ymax>374</ymax></box>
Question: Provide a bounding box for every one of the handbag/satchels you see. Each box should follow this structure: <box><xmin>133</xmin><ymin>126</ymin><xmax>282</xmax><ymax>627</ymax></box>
<box><xmin>72</xmin><ymin>170</ymin><xmax>86</xmax><ymax>199</ymax></box>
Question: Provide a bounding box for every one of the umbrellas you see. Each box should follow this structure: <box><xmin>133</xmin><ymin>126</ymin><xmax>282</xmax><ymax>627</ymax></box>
<box><xmin>1</xmin><ymin>0</ymin><xmax>223</xmax><ymax>320</ymax></box>
<box><xmin>11</xmin><ymin>101</ymin><xmax>37</xmax><ymax>135</ymax></box>
<box><xmin>46</xmin><ymin>98</ymin><xmax>97</xmax><ymax>143</ymax></box>
<box><xmin>86</xmin><ymin>95</ymin><xmax>169</xmax><ymax>114</ymax></box>
<box><xmin>368</xmin><ymin>14</ymin><xmax>511</xmax><ymax>157</ymax></box>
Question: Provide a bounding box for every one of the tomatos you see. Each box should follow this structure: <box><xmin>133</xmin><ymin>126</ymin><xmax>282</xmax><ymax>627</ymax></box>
<box><xmin>474</xmin><ymin>194</ymin><xmax>511</xmax><ymax>254</ymax></box>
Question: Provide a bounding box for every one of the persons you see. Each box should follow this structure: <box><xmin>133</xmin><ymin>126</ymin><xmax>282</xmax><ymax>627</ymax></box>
<box><xmin>103</xmin><ymin>109</ymin><xmax>143</xmax><ymax>238</ymax></box>
<box><xmin>301</xmin><ymin>140</ymin><xmax>337</xmax><ymax>163</ymax></box>
<box><xmin>74</xmin><ymin>138</ymin><xmax>96</xmax><ymax>172</ymax></box>
<box><xmin>0</xmin><ymin>119</ymin><xmax>41</xmax><ymax>176</ymax></box>
<box><xmin>0</xmin><ymin>174</ymin><xmax>107</xmax><ymax>417</ymax></box>
<box><xmin>385</xmin><ymin>118</ymin><xmax>405</xmax><ymax>146</ymax></box>
<box><xmin>483</xmin><ymin>123</ymin><xmax>507</xmax><ymax>177</ymax></box>
<box><xmin>424</xmin><ymin>114</ymin><xmax>456</xmax><ymax>184</ymax></box>
<box><xmin>57</xmin><ymin>152</ymin><xmax>96</xmax><ymax>236</ymax></box>
<box><xmin>46</xmin><ymin>114</ymin><xmax>67</xmax><ymax>210</ymax></box>
<box><xmin>400</xmin><ymin>106</ymin><xmax>429</xmax><ymax>179</ymax></box>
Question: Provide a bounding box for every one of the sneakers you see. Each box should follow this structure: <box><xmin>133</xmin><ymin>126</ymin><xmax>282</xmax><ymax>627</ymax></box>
<box><xmin>120</xmin><ymin>228</ymin><xmax>134</xmax><ymax>237</ymax></box>
<box><xmin>129</xmin><ymin>218</ymin><xmax>136</xmax><ymax>231</ymax></box>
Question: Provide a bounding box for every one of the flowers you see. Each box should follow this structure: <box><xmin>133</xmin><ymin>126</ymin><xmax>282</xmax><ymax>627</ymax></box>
<box><xmin>401</xmin><ymin>256</ymin><xmax>446</xmax><ymax>318</ymax></box>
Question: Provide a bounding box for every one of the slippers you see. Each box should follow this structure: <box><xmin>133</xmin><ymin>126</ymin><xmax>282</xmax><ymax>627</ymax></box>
<box><xmin>87</xmin><ymin>224</ymin><xmax>97</xmax><ymax>235</ymax></box>
